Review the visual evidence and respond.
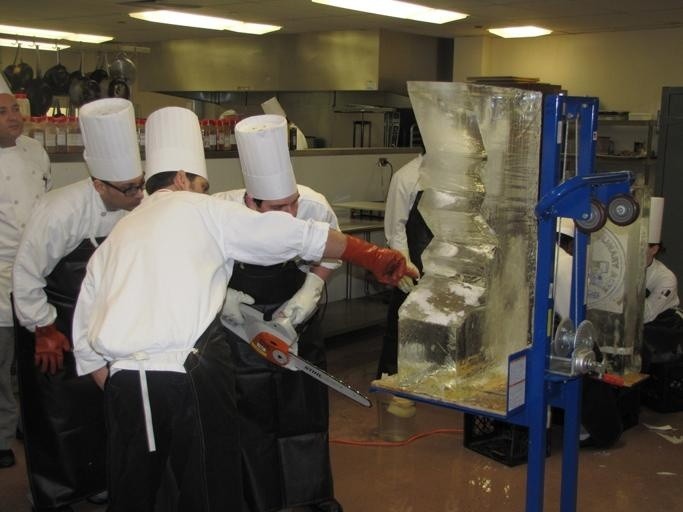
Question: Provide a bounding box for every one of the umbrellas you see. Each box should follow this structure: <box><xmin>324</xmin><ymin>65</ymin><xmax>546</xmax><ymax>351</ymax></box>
<box><xmin>0</xmin><ymin>448</ymin><xmax>15</xmax><ymax>468</ymax></box>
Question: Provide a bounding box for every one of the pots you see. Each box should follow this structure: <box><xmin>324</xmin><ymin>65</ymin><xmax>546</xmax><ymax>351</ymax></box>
<box><xmin>2</xmin><ymin>43</ymin><xmax>135</xmax><ymax>115</ymax></box>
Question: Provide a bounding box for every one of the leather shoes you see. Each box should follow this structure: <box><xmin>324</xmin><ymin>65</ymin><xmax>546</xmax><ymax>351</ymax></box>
<box><xmin>272</xmin><ymin>271</ymin><xmax>325</xmax><ymax>325</ymax></box>
<box><xmin>222</xmin><ymin>287</ymin><xmax>255</xmax><ymax>325</ymax></box>
<box><xmin>34</xmin><ymin>325</ymin><xmax>70</xmax><ymax>375</ymax></box>
<box><xmin>341</xmin><ymin>235</ymin><xmax>420</xmax><ymax>293</ymax></box>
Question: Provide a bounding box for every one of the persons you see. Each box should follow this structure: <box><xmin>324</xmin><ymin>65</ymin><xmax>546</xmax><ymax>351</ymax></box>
<box><xmin>377</xmin><ymin>151</ymin><xmax>434</xmax><ymax>381</ymax></box>
<box><xmin>71</xmin><ymin>171</ymin><xmax>417</xmax><ymax>512</ymax></box>
<box><xmin>553</xmin><ymin>233</ymin><xmax>574</xmax><ymax>340</ymax></box>
<box><xmin>210</xmin><ymin>184</ymin><xmax>343</xmax><ymax>512</ymax></box>
<box><xmin>11</xmin><ymin>172</ymin><xmax>145</xmax><ymax>512</ymax></box>
<box><xmin>0</xmin><ymin>94</ymin><xmax>52</xmax><ymax>468</ymax></box>
<box><xmin>642</xmin><ymin>243</ymin><xmax>683</xmax><ymax>351</ymax></box>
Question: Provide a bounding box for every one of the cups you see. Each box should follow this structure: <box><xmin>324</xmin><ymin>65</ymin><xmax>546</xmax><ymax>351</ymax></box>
<box><xmin>92</xmin><ymin>171</ymin><xmax>145</xmax><ymax>197</ymax></box>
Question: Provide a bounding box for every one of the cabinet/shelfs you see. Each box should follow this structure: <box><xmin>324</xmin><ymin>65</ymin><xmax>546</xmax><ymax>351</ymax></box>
<box><xmin>15</xmin><ymin>92</ymin><xmax>242</xmax><ymax>157</ymax></box>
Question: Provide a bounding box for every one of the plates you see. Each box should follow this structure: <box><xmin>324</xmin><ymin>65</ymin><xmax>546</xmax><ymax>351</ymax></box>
<box><xmin>317</xmin><ymin>201</ymin><xmax>388</xmax><ymax>340</ymax></box>
<box><xmin>653</xmin><ymin>87</ymin><xmax>683</xmax><ymax>310</ymax></box>
<box><xmin>560</xmin><ymin>120</ymin><xmax>653</xmax><ymax>185</ymax></box>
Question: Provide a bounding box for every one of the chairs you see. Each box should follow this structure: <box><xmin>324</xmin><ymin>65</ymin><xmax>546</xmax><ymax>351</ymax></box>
<box><xmin>16</xmin><ymin>424</ymin><xmax>24</xmax><ymax>440</ymax></box>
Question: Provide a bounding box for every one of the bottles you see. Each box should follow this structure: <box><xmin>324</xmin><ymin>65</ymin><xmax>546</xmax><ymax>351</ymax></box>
<box><xmin>78</xmin><ymin>97</ymin><xmax>209</xmax><ymax>188</ymax></box>
<box><xmin>646</xmin><ymin>195</ymin><xmax>664</xmax><ymax>245</ymax></box>
<box><xmin>219</xmin><ymin>109</ymin><xmax>236</xmax><ymax>120</ymax></box>
<box><xmin>0</xmin><ymin>73</ymin><xmax>13</xmax><ymax>95</ymax></box>
<box><xmin>234</xmin><ymin>97</ymin><xmax>297</xmax><ymax>200</ymax></box>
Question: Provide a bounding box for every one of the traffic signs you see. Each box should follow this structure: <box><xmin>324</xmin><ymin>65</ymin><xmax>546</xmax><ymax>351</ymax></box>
<box><xmin>379</xmin><ymin>157</ymin><xmax>387</xmax><ymax>167</ymax></box>
